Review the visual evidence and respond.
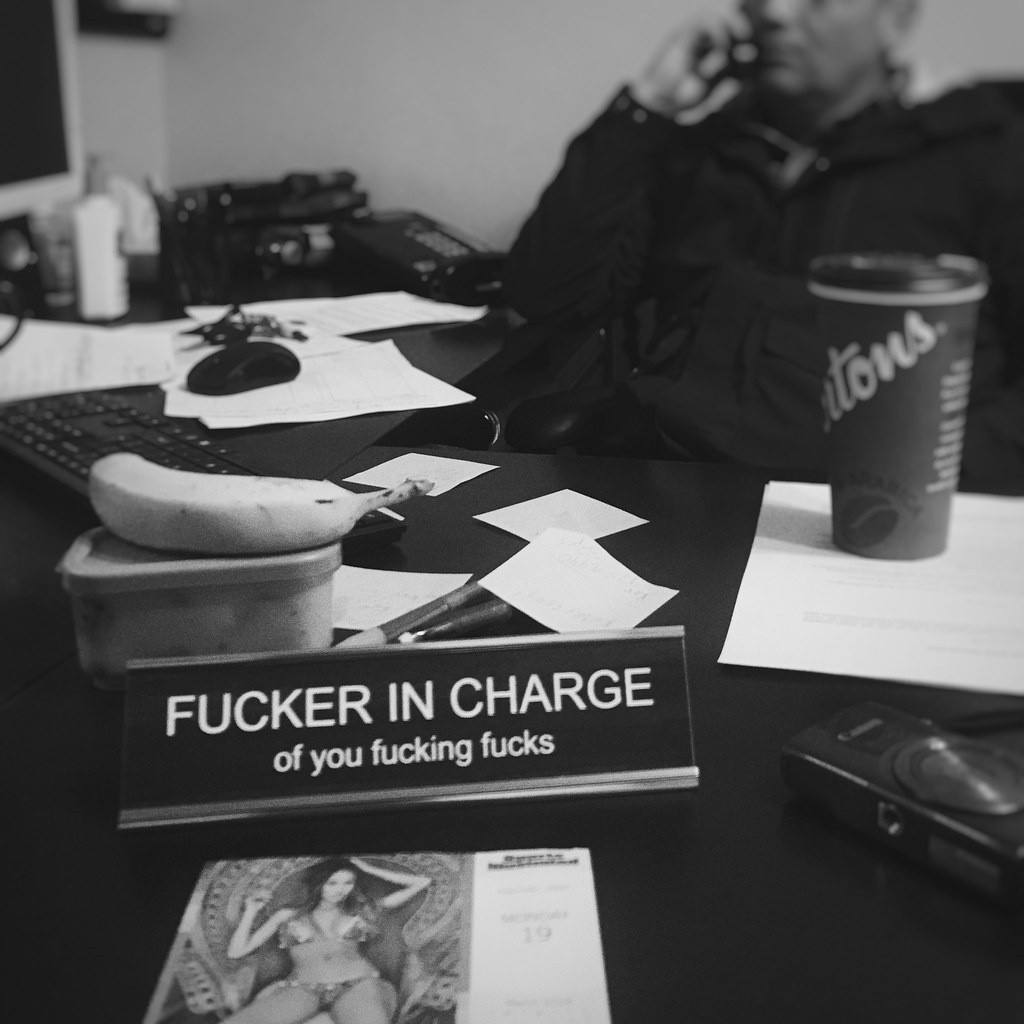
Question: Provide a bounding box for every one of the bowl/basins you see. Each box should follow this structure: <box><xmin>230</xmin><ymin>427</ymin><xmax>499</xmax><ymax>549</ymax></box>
<box><xmin>56</xmin><ymin>522</ymin><xmax>345</xmax><ymax>692</ymax></box>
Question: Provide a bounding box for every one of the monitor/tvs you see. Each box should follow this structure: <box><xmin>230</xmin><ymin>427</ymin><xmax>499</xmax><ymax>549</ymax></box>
<box><xmin>0</xmin><ymin>0</ymin><xmax>85</xmax><ymax>217</ymax></box>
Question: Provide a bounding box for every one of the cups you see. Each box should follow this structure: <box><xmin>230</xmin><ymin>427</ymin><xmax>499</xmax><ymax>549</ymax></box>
<box><xmin>806</xmin><ymin>252</ymin><xmax>988</xmax><ymax>556</ymax></box>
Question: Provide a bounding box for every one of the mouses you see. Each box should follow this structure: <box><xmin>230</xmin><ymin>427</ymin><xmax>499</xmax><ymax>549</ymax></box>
<box><xmin>187</xmin><ymin>342</ymin><xmax>302</xmax><ymax>395</ymax></box>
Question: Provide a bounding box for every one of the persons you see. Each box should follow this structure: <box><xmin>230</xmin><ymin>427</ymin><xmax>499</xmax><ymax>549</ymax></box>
<box><xmin>224</xmin><ymin>858</ymin><xmax>430</xmax><ymax>1024</ymax></box>
<box><xmin>499</xmin><ymin>0</ymin><xmax>1024</xmax><ymax>495</ymax></box>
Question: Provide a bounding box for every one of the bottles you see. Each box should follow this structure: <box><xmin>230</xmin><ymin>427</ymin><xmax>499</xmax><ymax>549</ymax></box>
<box><xmin>73</xmin><ymin>153</ymin><xmax>131</xmax><ymax>324</ymax></box>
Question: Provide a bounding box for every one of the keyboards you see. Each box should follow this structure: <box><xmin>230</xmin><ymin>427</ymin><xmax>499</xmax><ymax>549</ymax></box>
<box><xmin>0</xmin><ymin>391</ymin><xmax>407</xmax><ymax>558</ymax></box>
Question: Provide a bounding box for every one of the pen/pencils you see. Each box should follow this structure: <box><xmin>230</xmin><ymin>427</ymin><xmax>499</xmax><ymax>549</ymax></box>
<box><xmin>394</xmin><ymin>599</ymin><xmax>516</xmax><ymax>645</ymax></box>
<box><xmin>327</xmin><ymin>574</ymin><xmax>493</xmax><ymax>655</ymax></box>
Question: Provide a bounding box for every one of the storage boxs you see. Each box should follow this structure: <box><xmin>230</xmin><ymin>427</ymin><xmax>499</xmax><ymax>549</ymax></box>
<box><xmin>61</xmin><ymin>526</ymin><xmax>342</xmax><ymax>690</ymax></box>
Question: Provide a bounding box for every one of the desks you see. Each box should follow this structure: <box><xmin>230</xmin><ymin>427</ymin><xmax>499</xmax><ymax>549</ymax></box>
<box><xmin>0</xmin><ymin>253</ymin><xmax>1024</xmax><ymax>1024</ymax></box>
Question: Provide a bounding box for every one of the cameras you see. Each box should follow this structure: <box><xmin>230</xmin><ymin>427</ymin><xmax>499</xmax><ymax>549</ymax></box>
<box><xmin>787</xmin><ymin>703</ymin><xmax>1024</xmax><ymax>916</ymax></box>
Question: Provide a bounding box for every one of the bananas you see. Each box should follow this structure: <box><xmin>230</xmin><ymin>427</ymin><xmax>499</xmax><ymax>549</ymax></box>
<box><xmin>87</xmin><ymin>450</ymin><xmax>435</xmax><ymax>556</ymax></box>
